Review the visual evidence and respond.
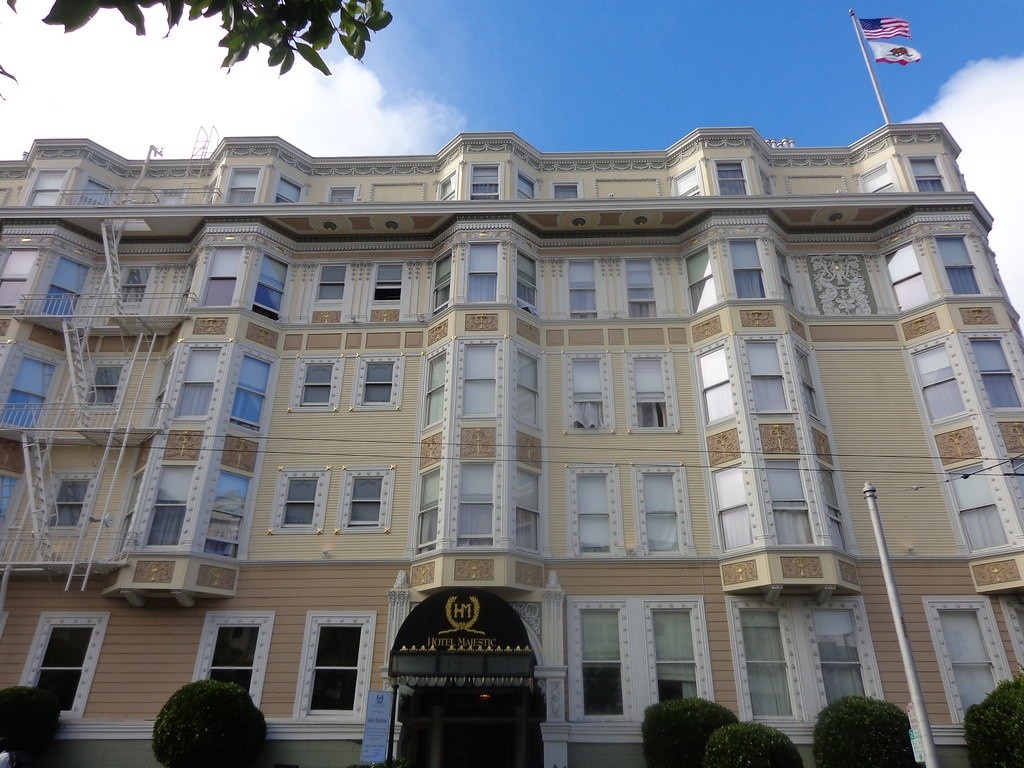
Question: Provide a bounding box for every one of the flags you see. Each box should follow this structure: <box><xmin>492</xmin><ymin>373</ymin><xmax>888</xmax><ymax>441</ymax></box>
<box><xmin>859</xmin><ymin>17</ymin><xmax>913</xmax><ymax>40</ymax></box>
<box><xmin>867</xmin><ymin>40</ymin><xmax>921</xmax><ymax>67</ymax></box>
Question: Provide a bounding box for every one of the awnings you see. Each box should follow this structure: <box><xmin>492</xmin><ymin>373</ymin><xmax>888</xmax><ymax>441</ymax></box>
<box><xmin>387</xmin><ymin>589</ymin><xmax>542</xmax><ymax>761</ymax></box>
